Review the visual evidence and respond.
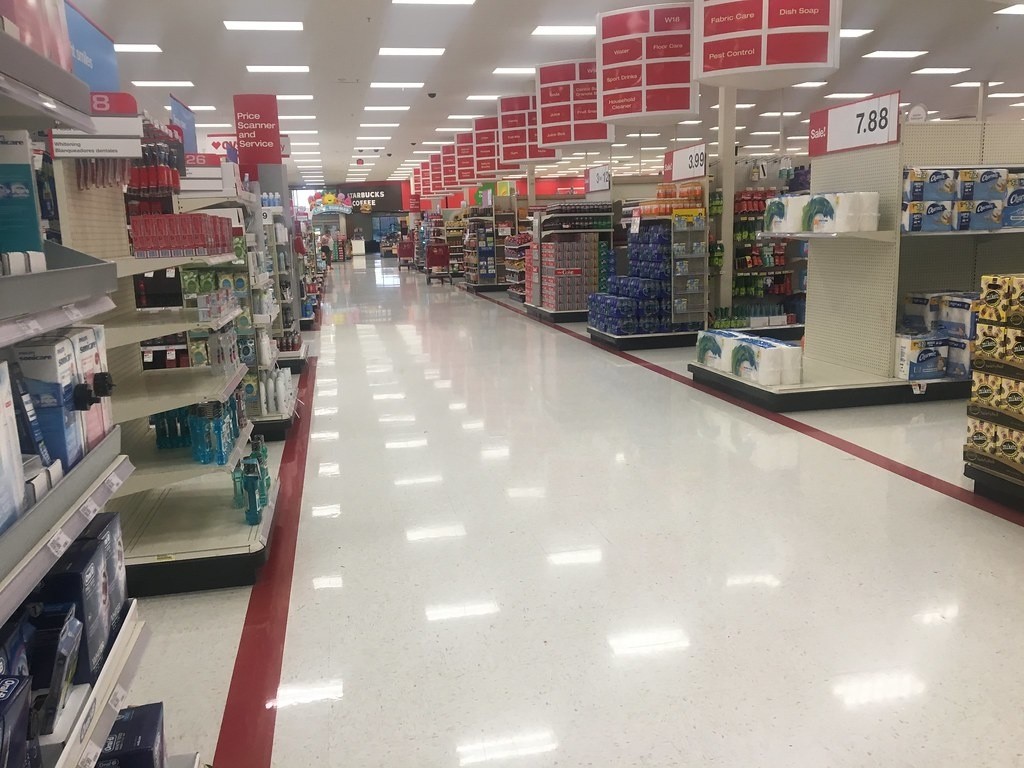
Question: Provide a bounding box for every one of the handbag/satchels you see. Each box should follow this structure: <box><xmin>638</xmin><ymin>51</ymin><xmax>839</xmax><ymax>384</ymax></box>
<box><xmin>321</xmin><ymin>246</ymin><xmax>329</xmax><ymax>252</ymax></box>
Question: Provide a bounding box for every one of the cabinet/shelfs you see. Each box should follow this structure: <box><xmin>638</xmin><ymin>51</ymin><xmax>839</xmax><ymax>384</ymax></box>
<box><xmin>50</xmin><ymin>135</ymin><xmax>282</xmax><ymax>599</ymax></box>
<box><xmin>692</xmin><ymin>110</ymin><xmax>1024</xmax><ymax>412</ymax></box>
<box><xmin>0</xmin><ymin>30</ymin><xmax>218</xmax><ymax>768</ymax></box>
<box><xmin>170</xmin><ymin>119</ymin><xmax>816</xmax><ymax>449</ymax></box>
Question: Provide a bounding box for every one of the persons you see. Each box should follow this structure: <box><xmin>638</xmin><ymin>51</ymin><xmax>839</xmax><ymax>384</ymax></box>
<box><xmin>322</xmin><ymin>230</ymin><xmax>334</xmax><ymax>271</ymax></box>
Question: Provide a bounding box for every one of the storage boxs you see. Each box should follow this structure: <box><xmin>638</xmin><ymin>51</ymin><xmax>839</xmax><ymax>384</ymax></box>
<box><xmin>893</xmin><ymin>273</ymin><xmax>1024</xmax><ymax>514</ymax></box>
<box><xmin>0</xmin><ymin>324</ymin><xmax>170</xmax><ymax>768</ymax></box>
<box><xmin>901</xmin><ymin>168</ymin><xmax>1024</xmax><ymax>233</ymax></box>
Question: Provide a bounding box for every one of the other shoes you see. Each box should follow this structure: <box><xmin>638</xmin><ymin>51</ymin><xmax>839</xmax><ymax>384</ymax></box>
<box><xmin>330</xmin><ymin>268</ymin><xmax>334</xmax><ymax>270</ymax></box>
